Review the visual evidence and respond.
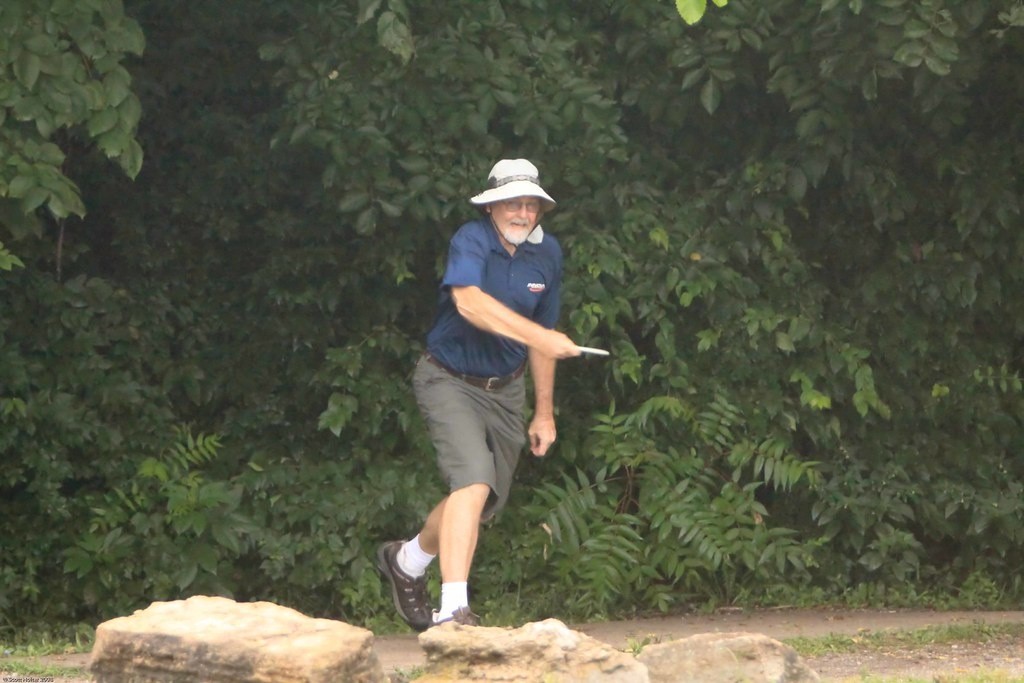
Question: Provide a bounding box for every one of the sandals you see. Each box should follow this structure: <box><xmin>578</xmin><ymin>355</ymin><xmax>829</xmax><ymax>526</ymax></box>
<box><xmin>432</xmin><ymin>607</ymin><xmax>480</xmax><ymax>627</ymax></box>
<box><xmin>378</xmin><ymin>539</ymin><xmax>434</xmax><ymax>632</ymax></box>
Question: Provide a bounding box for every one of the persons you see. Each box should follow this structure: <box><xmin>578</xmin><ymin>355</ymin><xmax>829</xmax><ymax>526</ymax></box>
<box><xmin>380</xmin><ymin>158</ymin><xmax>582</xmax><ymax>632</ymax></box>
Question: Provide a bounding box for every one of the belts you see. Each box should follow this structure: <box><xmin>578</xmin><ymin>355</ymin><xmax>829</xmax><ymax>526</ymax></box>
<box><xmin>423</xmin><ymin>352</ymin><xmax>525</xmax><ymax>391</ymax></box>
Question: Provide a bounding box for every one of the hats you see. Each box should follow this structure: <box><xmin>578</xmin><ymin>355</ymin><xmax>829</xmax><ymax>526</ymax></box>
<box><xmin>469</xmin><ymin>158</ymin><xmax>556</xmax><ymax>213</ymax></box>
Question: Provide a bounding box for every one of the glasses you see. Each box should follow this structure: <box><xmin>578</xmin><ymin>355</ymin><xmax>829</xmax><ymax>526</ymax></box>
<box><xmin>502</xmin><ymin>202</ymin><xmax>542</xmax><ymax>213</ymax></box>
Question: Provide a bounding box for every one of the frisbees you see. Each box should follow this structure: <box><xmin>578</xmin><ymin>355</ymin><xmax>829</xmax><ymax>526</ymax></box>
<box><xmin>579</xmin><ymin>346</ymin><xmax>609</xmax><ymax>355</ymax></box>
